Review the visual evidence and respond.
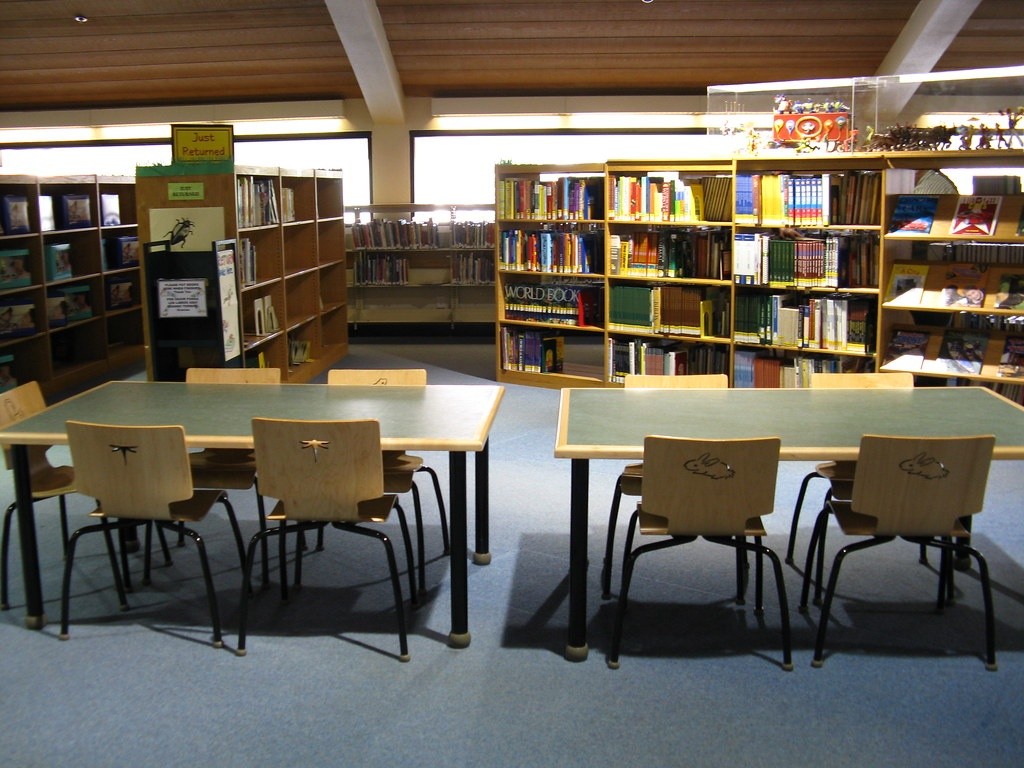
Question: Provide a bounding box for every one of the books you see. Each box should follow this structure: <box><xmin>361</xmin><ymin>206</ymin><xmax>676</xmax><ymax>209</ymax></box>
<box><xmin>236</xmin><ymin>175</ymin><xmax>311</xmax><ymax>377</ymax></box>
<box><xmin>453</xmin><ymin>253</ymin><xmax>496</xmax><ymax>288</ymax></box>
<box><xmin>609</xmin><ymin>174</ymin><xmax>734</xmax><ymax>385</ymax></box>
<box><xmin>500</xmin><ymin>178</ymin><xmax>604</xmax><ymax>375</ymax></box>
<box><xmin>731</xmin><ymin>167</ymin><xmax>882</xmax><ymax>389</ymax></box>
<box><xmin>453</xmin><ymin>220</ymin><xmax>496</xmax><ymax>249</ymax></box>
<box><xmin>884</xmin><ymin>169</ymin><xmax>1024</xmax><ymax>409</ymax></box>
<box><xmin>351</xmin><ymin>217</ymin><xmax>443</xmax><ymax>250</ymax></box>
<box><xmin>356</xmin><ymin>252</ymin><xmax>412</xmax><ymax>285</ymax></box>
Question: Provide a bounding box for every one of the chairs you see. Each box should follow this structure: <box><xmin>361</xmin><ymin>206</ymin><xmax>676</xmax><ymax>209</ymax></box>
<box><xmin>236</xmin><ymin>417</ymin><xmax>418</xmax><ymax>661</ymax></box>
<box><xmin>797</xmin><ymin>434</ymin><xmax>997</xmax><ymax>671</ymax></box>
<box><xmin>143</xmin><ymin>368</ymin><xmax>283</xmax><ymax>588</ymax></box>
<box><xmin>609</xmin><ymin>436</ymin><xmax>794</xmax><ymax>671</ymax></box>
<box><xmin>785</xmin><ymin>371</ymin><xmax>956</xmax><ymax>604</ymax></box>
<box><xmin>602</xmin><ymin>374</ymin><xmax>748</xmax><ymax>605</ymax></box>
<box><xmin>60</xmin><ymin>421</ymin><xmax>252</xmax><ymax>646</ymax></box>
<box><xmin>295</xmin><ymin>368</ymin><xmax>451</xmax><ymax>598</ymax></box>
<box><xmin>0</xmin><ymin>381</ymin><xmax>174</xmax><ymax>612</ymax></box>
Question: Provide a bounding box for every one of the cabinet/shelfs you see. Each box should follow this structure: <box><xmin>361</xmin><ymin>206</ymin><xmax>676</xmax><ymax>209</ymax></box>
<box><xmin>135</xmin><ymin>167</ymin><xmax>349</xmax><ymax>384</ymax></box>
<box><xmin>0</xmin><ymin>173</ymin><xmax>145</xmax><ymax>396</ymax></box>
<box><xmin>493</xmin><ymin>147</ymin><xmax>1024</xmax><ymax>410</ymax></box>
<box><xmin>345</xmin><ymin>205</ymin><xmax>494</xmax><ymax>287</ymax></box>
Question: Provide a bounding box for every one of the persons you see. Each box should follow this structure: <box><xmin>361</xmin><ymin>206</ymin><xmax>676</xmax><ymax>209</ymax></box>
<box><xmin>1</xmin><ymin>199</ymin><xmax>138</xmax><ymax>391</ymax></box>
<box><xmin>957</xmin><ymin>107</ymin><xmax>1024</xmax><ymax>150</ymax></box>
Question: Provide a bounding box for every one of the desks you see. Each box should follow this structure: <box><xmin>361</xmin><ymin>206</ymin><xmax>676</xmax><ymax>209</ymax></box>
<box><xmin>0</xmin><ymin>380</ymin><xmax>505</xmax><ymax>646</ymax></box>
<box><xmin>553</xmin><ymin>387</ymin><xmax>1024</xmax><ymax>662</ymax></box>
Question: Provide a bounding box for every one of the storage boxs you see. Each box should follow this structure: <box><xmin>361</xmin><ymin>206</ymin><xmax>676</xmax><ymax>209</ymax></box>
<box><xmin>1</xmin><ymin>195</ymin><xmax>140</xmax><ymax>393</ymax></box>
